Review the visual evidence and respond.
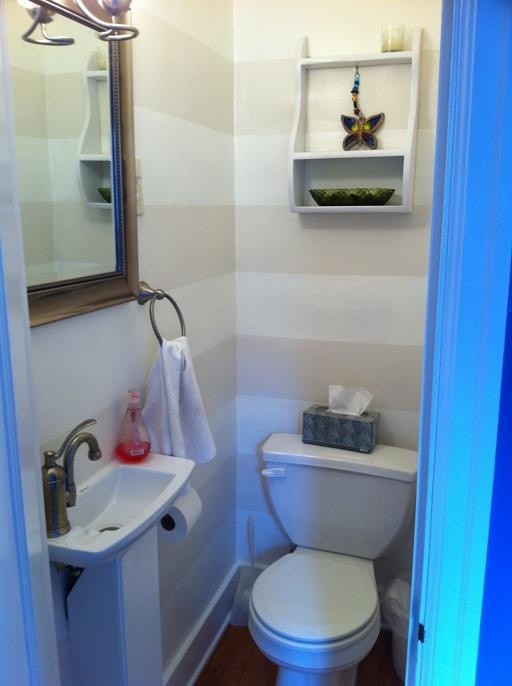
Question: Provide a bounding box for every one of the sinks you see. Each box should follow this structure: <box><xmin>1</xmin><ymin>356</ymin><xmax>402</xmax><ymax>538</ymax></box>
<box><xmin>45</xmin><ymin>452</ymin><xmax>195</xmax><ymax>570</ymax></box>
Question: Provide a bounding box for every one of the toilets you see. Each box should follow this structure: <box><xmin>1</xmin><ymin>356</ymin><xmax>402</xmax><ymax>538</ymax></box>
<box><xmin>250</xmin><ymin>435</ymin><xmax>424</xmax><ymax>685</ymax></box>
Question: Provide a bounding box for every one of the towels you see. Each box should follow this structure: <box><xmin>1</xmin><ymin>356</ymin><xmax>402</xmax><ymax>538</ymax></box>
<box><xmin>147</xmin><ymin>338</ymin><xmax>220</xmax><ymax>465</ymax></box>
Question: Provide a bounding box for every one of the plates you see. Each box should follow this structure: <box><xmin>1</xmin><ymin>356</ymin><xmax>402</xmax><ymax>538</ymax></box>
<box><xmin>97</xmin><ymin>185</ymin><xmax>113</xmax><ymax>203</ymax></box>
<box><xmin>310</xmin><ymin>188</ymin><xmax>396</xmax><ymax>206</ymax></box>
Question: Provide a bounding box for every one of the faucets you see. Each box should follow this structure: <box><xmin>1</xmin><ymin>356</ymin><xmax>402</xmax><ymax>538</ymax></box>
<box><xmin>41</xmin><ymin>419</ymin><xmax>101</xmax><ymax>538</ymax></box>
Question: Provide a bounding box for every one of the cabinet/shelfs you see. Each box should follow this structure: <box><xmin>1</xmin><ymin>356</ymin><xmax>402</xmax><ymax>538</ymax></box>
<box><xmin>76</xmin><ymin>49</ymin><xmax>113</xmax><ymax>211</ymax></box>
<box><xmin>287</xmin><ymin>26</ymin><xmax>421</xmax><ymax>214</ymax></box>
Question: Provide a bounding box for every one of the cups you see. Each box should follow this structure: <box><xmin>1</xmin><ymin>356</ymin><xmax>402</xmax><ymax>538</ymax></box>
<box><xmin>97</xmin><ymin>43</ymin><xmax>108</xmax><ymax>70</ymax></box>
<box><xmin>380</xmin><ymin>20</ymin><xmax>406</xmax><ymax>52</ymax></box>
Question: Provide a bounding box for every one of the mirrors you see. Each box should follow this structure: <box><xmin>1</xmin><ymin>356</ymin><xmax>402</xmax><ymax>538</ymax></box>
<box><xmin>1</xmin><ymin>0</ymin><xmax>141</xmax><ymax>329</ymax></box>
